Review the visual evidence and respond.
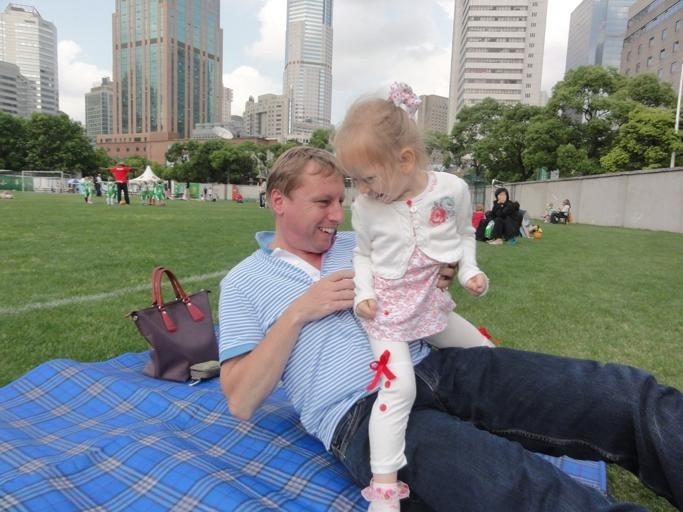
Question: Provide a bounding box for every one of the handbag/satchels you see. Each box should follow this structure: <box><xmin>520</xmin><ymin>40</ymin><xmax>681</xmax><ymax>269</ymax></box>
<box><xmin>130</xmin><ymin>266</ymin><xmax>220</xmax><ymax>382</ymax></box>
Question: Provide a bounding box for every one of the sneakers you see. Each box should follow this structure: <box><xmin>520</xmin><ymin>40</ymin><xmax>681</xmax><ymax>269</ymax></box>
<box><xmin>367</xmin><ymin>479</ymin><xmax>401</xmax><ymax>512</ymax></box>
<box><xmin>486</xmin><ymin>238</ymin><xmax>503</xmax><ymax>245</ymax></box>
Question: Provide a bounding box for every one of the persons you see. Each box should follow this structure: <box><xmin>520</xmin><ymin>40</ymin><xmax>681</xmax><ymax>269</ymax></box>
<box><xmin>217</xmin><ymin>146</ymin><xmax>682</xmax><ymax>512</ymax></box>
<box><xmin>551</xmin><ymin>199</ymin><xmax>570</xmax><ymax>224</ymax></box>
<box><xmin>257</xmin><ymin>178</ymin><xmax>267</xmax><ymax>208</ymax></box>
<box><xmin>111</xmin><ymin>163</ymin><xmax>134</xmax><ymax>205</ymax></box>
<box><xmin>78</xmin><ymin>173</ymin><xmax>102</xmax><ymax>204</ymax></box>
<box><xmin>334</xmin><ymin>83</ymin><xmax>499</xmax><ymax>512</ymax></box>
<box><xmin>472</xmin><ymin>188</ymin><xmax>523</xmax><ymax>245</ymax></box>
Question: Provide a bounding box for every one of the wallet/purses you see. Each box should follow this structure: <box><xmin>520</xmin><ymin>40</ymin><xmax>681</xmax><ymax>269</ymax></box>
<box><xmin>191</xmin><ymin>361</ymin><xmax>220</xmax><ymax>380</ymax></box>
